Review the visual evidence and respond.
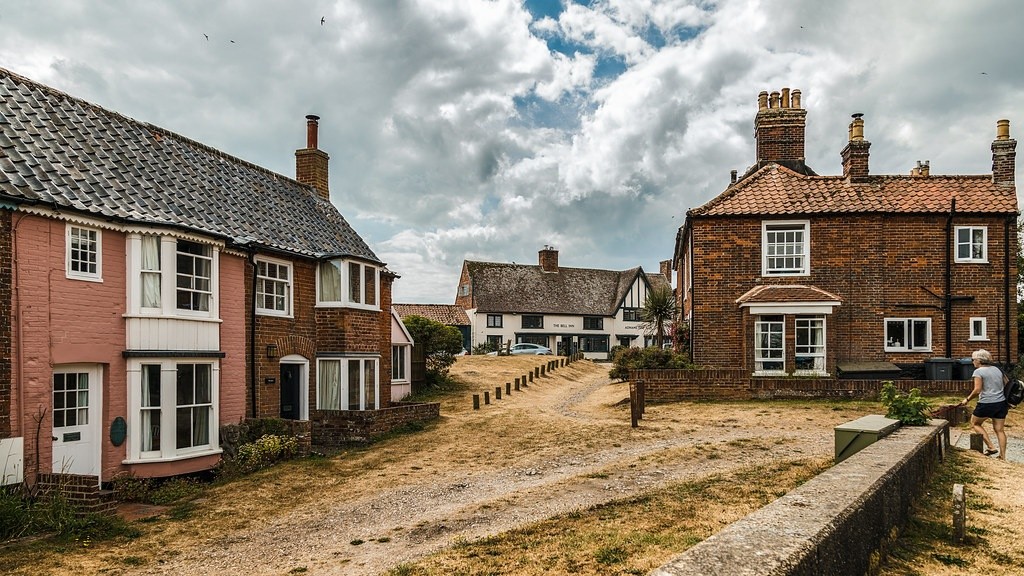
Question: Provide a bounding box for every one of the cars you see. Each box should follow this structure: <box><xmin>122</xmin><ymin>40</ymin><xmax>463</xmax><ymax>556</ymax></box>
<box><xmin>453</xmin><ymin>348</ymin><xmax>468</xmax><ymax>356</ymax></box>
<box><xmin>486</xmin><ymin>343</ymin><xmax>553</xmax><ymax>356</ymax></box>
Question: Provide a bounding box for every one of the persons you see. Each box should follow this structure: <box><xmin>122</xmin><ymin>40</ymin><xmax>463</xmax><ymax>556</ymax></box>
<box><xmin>962</xmin><ymin>349</ymin><xmax>1011</xmax><ymax>460</ymax></box>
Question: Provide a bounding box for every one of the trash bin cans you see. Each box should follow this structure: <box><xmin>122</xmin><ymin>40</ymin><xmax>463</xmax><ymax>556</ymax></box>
<box><xmin>926</xmin><ymin>356</ymin><xmax>974</xmax><ymax>379</ymax></box>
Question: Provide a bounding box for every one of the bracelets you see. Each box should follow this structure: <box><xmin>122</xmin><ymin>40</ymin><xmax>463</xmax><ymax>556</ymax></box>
<box><xmin>966</xmin><ymin>398</ymin><xmax>969</xmax><ymax>402</ymax></box>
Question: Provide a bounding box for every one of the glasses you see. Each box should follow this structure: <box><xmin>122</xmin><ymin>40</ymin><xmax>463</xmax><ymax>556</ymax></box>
<box><xmin>972</xmin><ymin>359</ymin><xmax>979</xmax><ymax>361</ymax></box>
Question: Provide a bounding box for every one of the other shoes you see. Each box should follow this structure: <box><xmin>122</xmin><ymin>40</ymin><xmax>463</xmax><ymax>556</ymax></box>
<box><xmin>996</xmin><ymin>456</ymin><xmax>1001</xmax><ymax>459</ymax></box>
<box><xmin>984</xmin><ymin>449</ymin><xmax>998</xmax><ymax>456</ymax></box>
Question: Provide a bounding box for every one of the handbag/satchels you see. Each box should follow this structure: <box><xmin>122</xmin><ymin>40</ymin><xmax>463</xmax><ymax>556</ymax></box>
<box><xmin>991</xmin><ymin>364</ymin><xmax>1024</xmax><ymax>409</ymax></box>
